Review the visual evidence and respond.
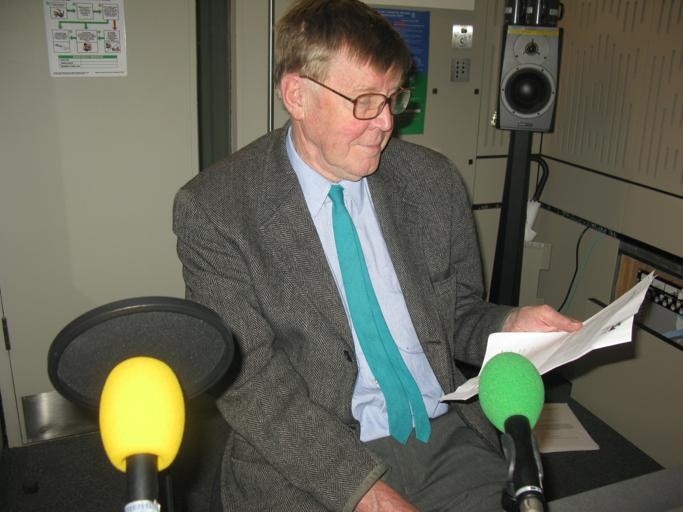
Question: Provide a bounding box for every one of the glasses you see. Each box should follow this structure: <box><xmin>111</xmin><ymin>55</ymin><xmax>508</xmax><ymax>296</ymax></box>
<box><xmin>296</xmin><ymin>74</ymin><xmax>411</xmax><ymax>119</ymax></box>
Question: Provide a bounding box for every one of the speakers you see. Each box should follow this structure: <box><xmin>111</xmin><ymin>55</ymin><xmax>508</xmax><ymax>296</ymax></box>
<box><xmin>496</xmin><ymin>25</ymin><xmax>564</xmax><ymax>134</ymax></box>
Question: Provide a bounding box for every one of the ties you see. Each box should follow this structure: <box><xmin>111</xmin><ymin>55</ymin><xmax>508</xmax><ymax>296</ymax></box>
<box><xmin>326</xmin><ymin>183</ymin><xmax>431</xmax><ymax>446</ymax></box>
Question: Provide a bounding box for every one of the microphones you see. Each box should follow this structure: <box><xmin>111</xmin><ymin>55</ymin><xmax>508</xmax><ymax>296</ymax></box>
<box><xmin>99</xmin><ymin>356</ymin><xmax>186</xmax><ymax>512</ymax></box>
<box><xmin>478</xmin><ymin>352</ymin><xmax>545</xmax><ymax>511</ymax></box>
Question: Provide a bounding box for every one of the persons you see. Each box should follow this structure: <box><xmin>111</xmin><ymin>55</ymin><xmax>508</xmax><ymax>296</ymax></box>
<box><xmin>170</xmin><ymin>0</ymin><xmax>585</xmax><ymax>512</ymax></box>
